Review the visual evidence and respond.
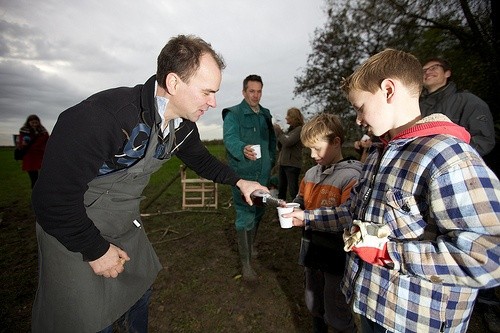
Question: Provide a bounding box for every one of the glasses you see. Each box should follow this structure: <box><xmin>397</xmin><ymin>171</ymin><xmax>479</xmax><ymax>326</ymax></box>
<box><xmin>423</xmin><ymin>64</ymin><xmax>446</xmax><ymax>74</ymax></box>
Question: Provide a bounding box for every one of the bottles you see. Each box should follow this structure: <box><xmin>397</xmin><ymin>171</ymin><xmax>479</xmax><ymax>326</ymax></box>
<box><xmin>241</xmin><ymin>189</ymin><xmax>285</xmax><ymax>207</ymax></box>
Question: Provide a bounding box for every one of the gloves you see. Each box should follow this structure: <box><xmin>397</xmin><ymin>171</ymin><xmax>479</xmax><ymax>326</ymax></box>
<box><xmin>342</xmin><ymin>219</ymin><xmax>393</xmax><ymax>272</ymax></box>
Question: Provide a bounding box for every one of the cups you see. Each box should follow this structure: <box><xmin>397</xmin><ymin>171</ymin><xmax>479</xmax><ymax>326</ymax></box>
<box><xmin>250</xmin><ymin>145</ymin><xmax>261</xmax><ymax>159</ymax></box>
<box><xmin>286</xmin><ymin>203</ymin><xmax>300</xmax><ymax>208</ymax></box>
<box><xmin>276</xmin><ymin>208</ymin><xmax>294</xmax><ymax>228</ymax></box>
<box><xmin>361</xmin><ymin>135</ymin><xmax>370</xmax><ymax>141</ymax></box>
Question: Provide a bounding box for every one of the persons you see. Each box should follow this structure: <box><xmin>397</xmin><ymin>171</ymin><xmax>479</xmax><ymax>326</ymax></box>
<box><xmin>31</xmin><ymin>33</ymin><xmax>270</xmax><ymax>333</ymax></box>
<box><xmin>222</xmin><ymin>75</ymin><xmax>277</xmax><ymax>285</ymax></box>
<box><xmin>13</xmin><ymin>115</ymin><xmax>50</xmax><ymax>190</ymax></box>
<box><xmin>273</xmin><ymin>48</ymin><xmax>500</xmax><ymax>333</ymax></box>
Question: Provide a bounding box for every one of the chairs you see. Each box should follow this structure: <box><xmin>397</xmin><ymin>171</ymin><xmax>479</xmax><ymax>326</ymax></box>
<box><xmin>179</xmin><ymin>164</ymin><xmax>220</xmax><ymax>211</ymax></box>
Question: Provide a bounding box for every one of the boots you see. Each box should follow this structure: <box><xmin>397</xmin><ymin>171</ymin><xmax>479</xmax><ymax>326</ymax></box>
<box><xmin>236</xmin><ymin>224</ymin><xmax>258</xmax><ymax>280</ymax></box>
